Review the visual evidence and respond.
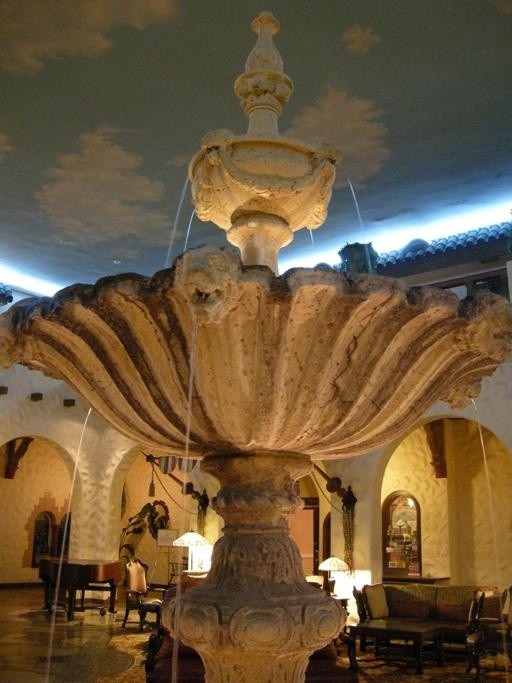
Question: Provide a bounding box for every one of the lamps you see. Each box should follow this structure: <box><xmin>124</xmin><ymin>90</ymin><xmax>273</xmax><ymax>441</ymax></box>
<box><xmin>173</xmin><ymin>528</ymin><xmax>210</xmax><ymax>570</ymax></box>
<box><xmin>317</xmin><ymin>556</ymin><xmax>349</xmax><ymax>596</ymax></box>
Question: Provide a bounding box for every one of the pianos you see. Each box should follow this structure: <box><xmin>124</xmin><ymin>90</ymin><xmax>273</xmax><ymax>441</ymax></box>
<box><xmin>39</xmin><ymin>558</ymin><xmax>121</xmax><ymax>622</ymax></box>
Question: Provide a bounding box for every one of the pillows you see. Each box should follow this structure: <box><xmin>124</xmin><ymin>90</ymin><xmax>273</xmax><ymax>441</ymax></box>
<box><xmin>361</xmin><ymin>583</ymin><xmax>467</xmax><ymax>623</ymax></box>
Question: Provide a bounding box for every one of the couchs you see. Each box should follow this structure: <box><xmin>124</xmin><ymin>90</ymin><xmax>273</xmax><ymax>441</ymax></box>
<box><xmin>353</xmin><ymin>580</ymin><xmax>509</xmax><ymax>671</ymax></box>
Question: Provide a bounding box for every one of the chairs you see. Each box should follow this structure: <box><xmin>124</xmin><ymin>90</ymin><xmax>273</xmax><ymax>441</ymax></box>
<box><xmin>123</xmin><ymin>558</ymin><xmax>162</xmax><ymax>631</ymax></box>
